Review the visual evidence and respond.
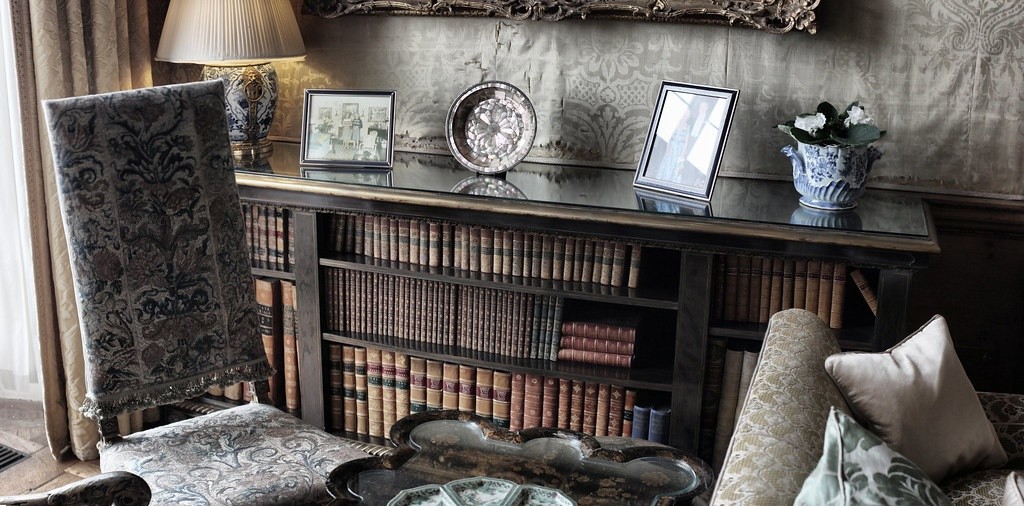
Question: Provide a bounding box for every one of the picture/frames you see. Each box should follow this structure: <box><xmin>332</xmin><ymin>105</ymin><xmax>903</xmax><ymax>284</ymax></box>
<box><xmin>299</xmin><ymin>87</ymin><xmax>397</xmax><ymax>169</ymax></box>
<box><xmin>300</xmin><ymin>166</ymin><xmax>395</xmax><ymax>188</ymax></box>
<box><xmin>631</xmin><ymin>79</ymin><xmax>741</xmax><ymax>201</ymax></box>
<box><xmin>633</xmin><ymin>187</ymin><xmax>714</xmax><ymax>218</ymax></box>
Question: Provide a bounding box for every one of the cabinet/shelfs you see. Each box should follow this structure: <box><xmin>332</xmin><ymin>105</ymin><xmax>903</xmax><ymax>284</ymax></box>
<box><xmin>158</xmin><ymin>171</ymin><xmax>919</xmax><ymax>485</ymax></box>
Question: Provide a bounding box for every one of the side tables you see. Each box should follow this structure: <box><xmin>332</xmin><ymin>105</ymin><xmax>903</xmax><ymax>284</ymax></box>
<box><xmin>321</xmin><ymin>407</ymin><xmax>715</xmax><ymax>506</ymax></box>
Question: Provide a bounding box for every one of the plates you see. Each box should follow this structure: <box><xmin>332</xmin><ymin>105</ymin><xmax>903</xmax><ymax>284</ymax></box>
<box><xmin>445</xmin><ymin>81</ymin><xmax>536</xmax><ymax>173</ymax></box>
<box><xmin>451</xmin><ymin>176</ymin><xmax>527</xmax><ymax>199</ymax></box>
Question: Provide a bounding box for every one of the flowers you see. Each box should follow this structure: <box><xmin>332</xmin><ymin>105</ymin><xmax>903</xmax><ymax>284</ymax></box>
<box><xmin>774</xmin><ymin>98</ymin><xmax>889</xmax><ymax>146</ymax></box>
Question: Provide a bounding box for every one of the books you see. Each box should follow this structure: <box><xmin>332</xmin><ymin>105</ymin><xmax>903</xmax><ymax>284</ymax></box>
<box><xmin>701</xmin><ymin>244</ymin><xmax>877</xmax><ymax>486</ymax></box>
<box><xmin>316</xmin><ymin>214</ymin><xmax>676</xmax><ymax>469</ymax></box>
<box><xmin>190</xmin><ymin>197</ymin><xmax>297</xmax><ymax>421</ymax></box>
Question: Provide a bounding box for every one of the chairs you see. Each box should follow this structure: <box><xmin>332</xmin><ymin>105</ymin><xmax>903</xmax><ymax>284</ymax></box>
<box><xmin>41</xmin><ymin>77</ymin><xmax>373</xmax><ymax>506</ymax></box>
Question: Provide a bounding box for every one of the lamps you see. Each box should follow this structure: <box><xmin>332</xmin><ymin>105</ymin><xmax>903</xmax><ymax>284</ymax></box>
<box><xmin>154</xmin><ymin>0</ymin><xmax>306</xmax><ymax>153</ymax></box>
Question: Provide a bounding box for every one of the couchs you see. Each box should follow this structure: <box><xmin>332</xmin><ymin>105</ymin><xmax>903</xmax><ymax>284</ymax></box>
<box><xmin>707</xmin><ymin>307</ymin><xmax>1024</xmax><ymax>506</ymax></box>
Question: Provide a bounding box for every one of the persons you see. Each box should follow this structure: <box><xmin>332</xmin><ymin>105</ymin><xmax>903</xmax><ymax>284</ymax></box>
<box><xmin>655</xmin><ymin>102</ymin><xmax>694</xmax><ymax>184</ymax></box>
<box><xmin>342</xmin><ymin>112</ymin><xmax>352</xmax><ymax>150</ymax></box>
<box><xmin>676</xmin><ymin>101</ymin><xmax>713</xmax><ymax>185</ymax></box>
<box><xmin>352</xmin><ymin>112</ymin><xmax>364</xmax><ymax>151</ymax></box>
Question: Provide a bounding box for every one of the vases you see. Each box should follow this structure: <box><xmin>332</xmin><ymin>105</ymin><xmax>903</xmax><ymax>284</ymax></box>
<box><xmin>782</xmin><ymin>139</ymin><xmax>883</xmax><ymax>211</ymax></box>
<box><xmin>789</xmin><ymin>203</ymin><xmax>864</xmax><ymax>232</ymax></box>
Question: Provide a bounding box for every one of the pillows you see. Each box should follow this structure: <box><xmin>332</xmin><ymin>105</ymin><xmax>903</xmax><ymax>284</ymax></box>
<box><xmin>824</xmin><ymin>313</ymin><xmax>1009</xmax><ymax>487</ymax></box>
<box><xmin>790</xmin><ymin>404</ymin><xmax>956</xmax><ymax>505</ymax></box>
